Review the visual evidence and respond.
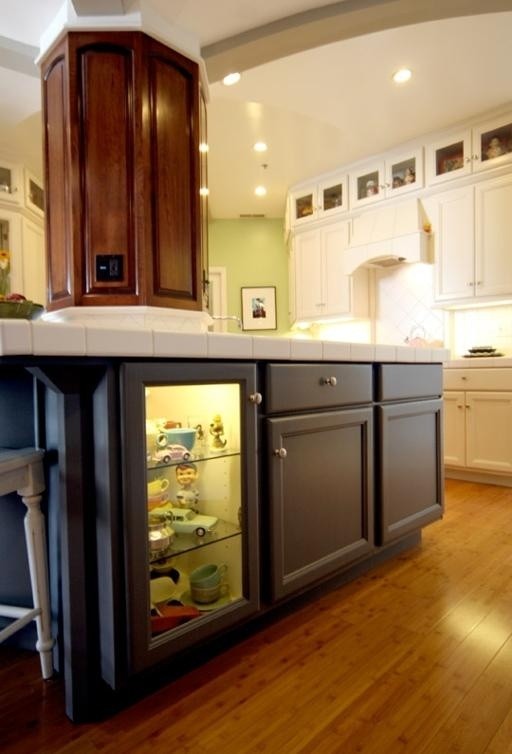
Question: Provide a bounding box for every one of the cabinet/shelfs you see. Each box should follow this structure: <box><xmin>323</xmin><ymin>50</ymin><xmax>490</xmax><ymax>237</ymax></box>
<box><xmin>265</xmin><ymin>363</ymin><xmax>373</xmax><ymax>601</ymax></box>
<box><xmin>444</xmin><ymin>367</ymin><xmax>512</xmax><ymax>474</ymax></box>
<box><xmin>119</xmin><ymin>361</ymin><xmax>265</xmax><ymax>673</ymax></box>
<box><xmin>377</xmin><ymin>364</ymin><xmax>445</xmax><ymax>545</ymax></box>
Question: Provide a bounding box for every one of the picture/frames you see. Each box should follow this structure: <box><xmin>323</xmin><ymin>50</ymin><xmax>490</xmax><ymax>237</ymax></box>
<box><xmin>240</xmin><ymin>286</ymin><xmax>278</xmax><ymax>331</ymax></box>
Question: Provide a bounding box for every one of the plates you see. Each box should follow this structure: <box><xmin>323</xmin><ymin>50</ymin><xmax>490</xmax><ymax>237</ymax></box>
<box><xmin>464</xmin><ymin>344</ymin><xmax>506</xmax><ymax>358</ymax></box>
<box><xmin>0</xmin><ymin>298</ymin><xmax>44</xmax><ymax>320</ymax></box>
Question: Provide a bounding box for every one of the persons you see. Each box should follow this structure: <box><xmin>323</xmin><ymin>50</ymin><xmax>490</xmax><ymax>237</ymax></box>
<box><xmin>176</xmin><ymin>463</ymin><xmax>199</xmax><ymax>514</ymax></box>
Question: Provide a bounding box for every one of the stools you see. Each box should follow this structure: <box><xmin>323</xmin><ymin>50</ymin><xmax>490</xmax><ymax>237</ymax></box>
<box><xmin>0</xmin><ymin>448</ymin><xmax>54</xmax><ymax>678</ymax></box>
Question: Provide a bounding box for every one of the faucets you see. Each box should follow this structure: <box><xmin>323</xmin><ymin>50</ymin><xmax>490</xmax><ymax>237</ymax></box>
<box><xmin>212</xmin><ymin>315</ymin><xmax>242</xmax><ymax>330</ymax></box>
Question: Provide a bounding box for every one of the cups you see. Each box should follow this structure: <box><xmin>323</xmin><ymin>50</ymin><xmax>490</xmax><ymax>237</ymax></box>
<box><xmin>143</xmin><ymin>413</ymin><xmax>229</xmax><ymax>607</ymax></box>
